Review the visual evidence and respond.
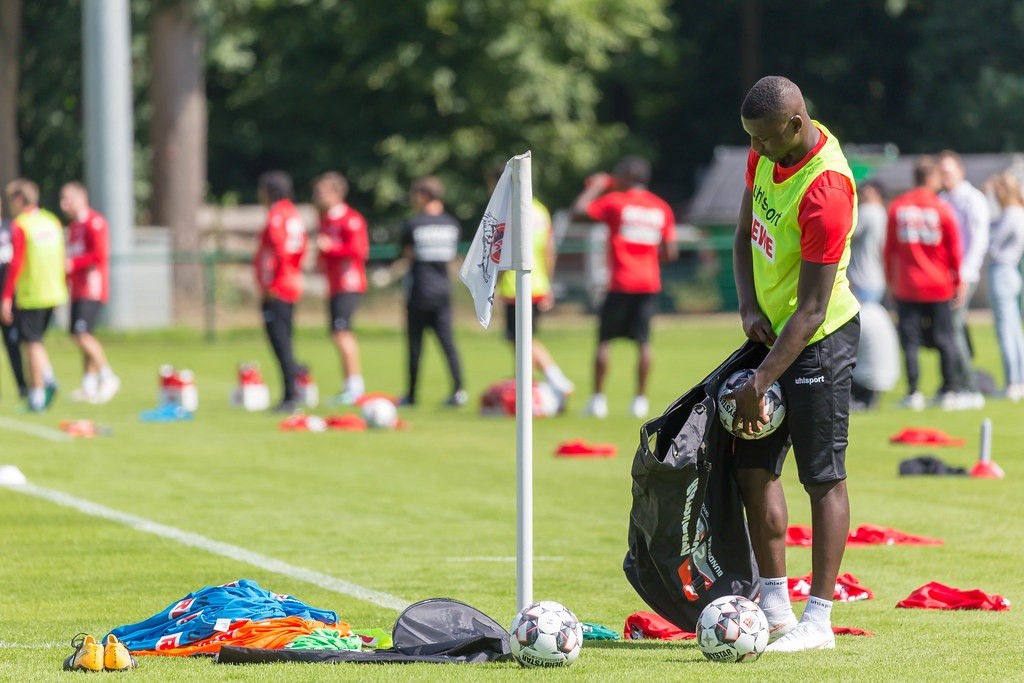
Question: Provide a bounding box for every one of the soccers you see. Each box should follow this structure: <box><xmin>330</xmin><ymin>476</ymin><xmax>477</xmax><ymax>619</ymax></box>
<box><xmin>717</xmin><ymin>369</ymin><xmax>787</xmax><ymax>441</ymax></box>
<box><xmin>363</xmin><ymin>397</ymin><xmax>398</xmax><ymax>431</ymax></box>
<box><xmin>507</xmin><ymin>602</ymin><xmax>584</xmax><ymax>668</ymax></box>
<box><xmin>696</xmin><ymin>594</ymin><xmax>771</xmax><ymax>662</ymax></box>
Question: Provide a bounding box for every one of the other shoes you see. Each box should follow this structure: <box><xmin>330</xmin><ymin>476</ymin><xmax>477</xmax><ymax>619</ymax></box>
<box><xmin>933</xmin><ymin>390</ymin><xmax>984</xmax><ymax>409</ymax></box>
<box><xmin>586</xmin><ymin>399</ymin><xmax>607</xmax><ymax>419</ymax></box>
<box><xmin>75</xmin><ymin>371</ymin><xmax>119</xmax><ymax>404</ymax></box>
<box><xmin>895</xmin><ymin>390</ymin><xmax>926</xmax><ymax>410</ymax></box>
<box><xmin>633</xmin><ymin>398</ymin><xmax>648</xmax><ymax>419</ymax></box>
<box><xmin>546</xmin><ymin>367</ymin><xmax>576</xmax><ymax>396</ymax></box>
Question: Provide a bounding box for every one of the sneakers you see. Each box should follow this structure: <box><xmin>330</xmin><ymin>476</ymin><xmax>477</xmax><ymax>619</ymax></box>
<box><xmin>767</xmin><ymin>616</ymin><xmax>798</xmax><ymax>634</ymax></box>
<box><xmin>62</xmin><ymin>633</ymin><xmax>104</xmax><ymax>672</ymax></box>
<box><xmin>104</xmin><ymin>634</ymin><xmax>137</xmax><ymax>670</ymax></box>
<box><xmin>765</xmin><ymin>623</ymin><xmax>835</xmax><ymax>653</ymax></box>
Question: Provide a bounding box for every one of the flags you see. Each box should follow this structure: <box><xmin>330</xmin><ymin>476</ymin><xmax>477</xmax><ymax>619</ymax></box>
<box><xmin>460</xmin><ymin>158</ymin><xmax>511</xmax><ymax>329</ymax></box>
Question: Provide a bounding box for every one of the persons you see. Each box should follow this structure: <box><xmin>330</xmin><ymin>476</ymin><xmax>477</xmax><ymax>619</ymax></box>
<box><xmin>846</xmin><ymin>177</ymin><xmax>888</xmax><ymax>407</ymax></box>
<box><xmin>1</xmin><ymin>178</ymin><xmax>69</xmax><ymax>409</ymax></box>
<box><xmin>495</xmin><ymin>194</ymin><xmax>575</xmax><ymax>399</ymax></box>
<box><xmin>568</xmin><ymin>152</ymin><xmax>680</xmax><ymax>418</ymax></box>
<box><xmin>253</xmin><ymin>171</ymin><xmax>306</xmax><ymax>411</ymax></box>
<box><xmin>394</xmin><ymin>175</ymin><xmax>467</xmax><ymax>406</ymax></box>
<box><xmin>883</xmin><ymin>156</ymin><xmax>966</xmax><ymax>408</ymax></box>
<box><xmin>985</xmin><ymin>171</ymin><xmax>1024</xmax><ymax>401</ymax></box>
<box><xmin>719</xmin><ymin>76</ymin><xmax>863</xmax><ymax>651</ymax></box>
<box><xmin>58</xmin><ymin>180</ymin><xmax>121</xmax><ymax>404</ymax></box>
<box><xmin>305</xmin><ymin>173</ymin><xmax>371</xmax><ymax>404</ymax></box>
<box><xmin>935</xmin><ymin>148</ymin><xmax>989</xmax><ymax>408</ymax></box>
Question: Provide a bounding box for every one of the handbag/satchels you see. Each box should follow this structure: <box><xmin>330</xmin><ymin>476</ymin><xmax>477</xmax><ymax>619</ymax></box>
<box><xmin>624</xmin><ymin>339</ymin><xmax>773</xmax><ymax>635</ymax></box>
